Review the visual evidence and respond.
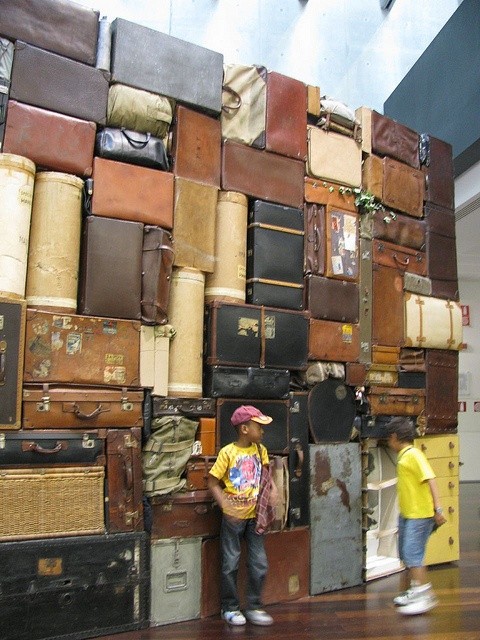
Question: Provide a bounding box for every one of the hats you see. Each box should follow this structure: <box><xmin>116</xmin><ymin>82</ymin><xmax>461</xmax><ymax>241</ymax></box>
<box><xmin>230</xmin><ymin>405</ymin><xmax>273</xmax><ymax>426</ymax></box>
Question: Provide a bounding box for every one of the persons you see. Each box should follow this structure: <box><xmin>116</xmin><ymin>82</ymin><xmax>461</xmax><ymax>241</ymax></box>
<box><xmin>206</xmin><ymin>405</ymin><xmax>274</xmax><ymax>627</ymax></box>
<box><xmin>385</xmin><ymin>416</ymin><xmax>446</xmax><ymax>615</ymax></box>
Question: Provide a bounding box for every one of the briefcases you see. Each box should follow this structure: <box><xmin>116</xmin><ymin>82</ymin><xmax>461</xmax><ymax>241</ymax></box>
<box><xmin>152</xmin><ymin>336</ymin><xmax>169</xmax><ymax>396</ymax></box>
<box><xmin>201</xmin><ymin>417</ymin><xmax>217</xmax><ymax>454</ymax></box>
<box><xmin>187</xmin><ymin>462</ymin><xmax>225</xmax><ymax>490</ymax></box>
<box><xmin>152</xmin><ymin>396</ymin><xmax>217</xmax><ymax>418</ymax></box>
<box><xmin>360</xmin><ymin>414</ymin><xmax>416</xmax><ymax>439</ymax></box>
<box><xmin>139</xmin><ymin>327</ymin><xmax>155</xmax><ymax>388</ymax></box>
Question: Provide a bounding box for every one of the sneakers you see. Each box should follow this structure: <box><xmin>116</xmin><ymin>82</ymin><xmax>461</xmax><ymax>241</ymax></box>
<box><xmin>246</xmin><ymin>609</ymin><xmax>274</xmax><ymax>625</ymax></box>
<box><xmin>220</xmin><ymin>609</ymin><xmax>247</xmax><ymax>626</ymax></box>
<box><xmin>392</xmin><ymin>589</ymin><xmax>414</xmax><ymax>605</ymax></box>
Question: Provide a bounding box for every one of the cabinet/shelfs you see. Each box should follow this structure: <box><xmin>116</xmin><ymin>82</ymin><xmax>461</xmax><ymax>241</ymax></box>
<box><xmin>364</xmin><ymin>439</ymin><xmax>407</xmax><ymax>582</ymax></box>
<box><xmin>413</xmin><ymin>434</ymin><xmax>460</xmax><ymax>568</ymax></box>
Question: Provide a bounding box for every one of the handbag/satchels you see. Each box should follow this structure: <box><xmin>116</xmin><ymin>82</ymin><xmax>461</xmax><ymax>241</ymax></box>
<box><xmin>107</xmin><ymin>83</ymin><xmax>173</xmax><ymax>140</ymax></box>
<box><xmin>141</xmin><ymin>223</ymin><xmax>176</xmax><ymax>326</ymax></box>
<box><xmin>254</xmin><ymin>465</ymin><xmax>277</xmax><ymax>537</ymax></box>
<box><xmin>297</xmin><ymin>360</ymin><xmax>345</xmax><ymax>384</ymax></box>
<box><xmin>141</xmin><ymin>415</ymin><xmax>200</xmax><ymax>498</ymax></box>
<box><xmin>95</xmin><ymin>126</ymin><xmax>170</xmax><ymax>171</ymax></box>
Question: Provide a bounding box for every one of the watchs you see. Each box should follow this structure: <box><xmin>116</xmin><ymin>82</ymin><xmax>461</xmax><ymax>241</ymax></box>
<box><xmin>434</xmin><ymin>507</ymin><xmax>442</xmax><ymax>514</ymax></box>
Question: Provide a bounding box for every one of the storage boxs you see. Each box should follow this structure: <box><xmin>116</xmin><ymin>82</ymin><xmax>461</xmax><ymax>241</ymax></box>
<box><xmin>204</xmin><ymin>299</ymin><xmax>309</xmax><ymax>372</ymax></box>
<box><xmin>143</xmin><ymin>224</ymin><xmax>171</xmax><ymax>325</ymax></box>
<box><xmin>308</xmin><ymin>319</ymin><xmax>362</xmax><ymax>363</ymax></box>
<box><xmin>3</xmin><ymin>101</ymin><xmax>96</xmax><ymax>177</ymax></box>
<box><xmin>150</xmin><ymin>496</ymin><xmax>221</xmax><ymax>537</ymax></box>
<box><xmin>365</xmin><ymin>363</ymin><xmax>400</xmax><ymax>387</ymax></box>
<box><xmin>404</xmin><ymin>271</ymin><xmax>432</xmax><ymax>297</ymax></box>
<box><xmin>371</xmin><ymin>266</ymin><xmax>403</xmax><ymax>348</ymax></box>
<box><xmin>172</xmin><ymin>176</ymin><xmax>218</xmax><ymax>274</ymax></box>
<box><xmin>184</xmin><ymin>462</ymin><xmax>212</xmax><ymax>493</ymax></box>
<box><xmin>364</xmin><ymin>153</ymin><xmax>383</xmax><ymax>201</ymax></box>
<box><xmin>383</xmin><ymin>155</ymin><xmax>425</xmax><ymax>219</ymax></box>
<box><xmin>150</xmin><ymin>538</ymin><xmax>202</xmax><ymax>626</ymax></box>
<box><xmin>24</xmin><ymin>308</ymin><xmax>141</xmax><ymax>390</ymax></box>
<box><xmin>304</xmin><ymin>274</ymin><xmax>359</xmax><ymax>323</ymax></box>
<box><xmin>306</xmin><ymin>85</ymin><xmax>321</xmax><ymax>119</ymax></box>
<box><xmin>0</xmin><ymin>35</ymin><xmax>14</xmax><ymax>155</ymax></box>
<box><xmin>203</xmin><ymin>529</ymin><xmax>311</xmax><ymax>618</ymax></box>
<box><xmin>169</xmin><ymin>103</ymin><xmax>220</xmax><ymax>187</ymax></box>
<box><xmin>10</xmin><ymin>38</ymin><xmax>110</xmax><ymax>127</ymax></box>
<box><xmin>195</xmin><ymin>416</ymin><xmax>217</xmax><ymax>458</ymax></box>
<box><xmin>90</xmin><ymin>155</ymin><xmax>174</xmax><ymax>230</ymax></box>
<box><xmin>111</xmin><ymin>18</ymin><xmax>223</xmax><ymax>117</ymax></box>
<box><xmin>371</xmin><ymin>110</ymin><xmax>423</xmax><ymax>170</ymax></box>
<box><xmin>0</xmin><ymin>298</ymin><xmax>28</xmax><ymax>430</ymax></box>
<box><xmin>77</xmin><ymin>214</ymin><xmax>144</xmax><ymax>320</ymax></box>
<box><xmin>246</xmin><ymin>199</ymin><xmax>305</xmax><ymax>310</ymax></box>
<box><xmin>432</xmin><ymin>279</ymin><xmax>460</xmax><ymax>301</ymax></box>
<box><xmin>372</xmin><ymin>207</ymin><xmax>427</xmax><ymax>251</ymax></box>
<box><xmin>0</xmin><ymin>0</ymin><xmax>99</xmax><ymax>68</ymax></box>
<box><xmin>1</xmin><ymin>532</ymin><xmax>147</xmax><ymax>640</ymax></box>
<box><xmin>221</xmin><ymin>139</ymin><xmax>305</xmax><ymax>211</ymax></box>
<box><xmin>216</xmin><ymin>397</ymin><xmax>289</xmax><ymax>456</ymax></box>
<box><xmin>423</xmin><ymin>200</ymin><xmax>458</xmax><ymax>283</ymax></box>
<box><xmin>343</xmin><ymin>363</ymin><xmax>365</xmax><ymax>386</ymax></box>
<box><xmin>266</xmin><ymin>70</ymin><xmax>308</xmax><ymax>162</ymax></box>
<box><xmin>309</xmin><ymin>442</ymin><xmax>365</xmax><ymax>595</ymax></box>
<box><xmin>400</xmin><ymin>348</ymin><xmax>426</xmax><ymax>372</ymax></box>
<box><xmin>425</xmin><ymin>346</ymin><xmax>459</xmax><ymax>435</ymax></box>
<box><xmin>398</xmin><ymin>370</ymin><xmax>426</xmax><ymax>389</ymax></box>
<box><xmin>403</xmin><ymin>290</ymin><xmax>463</xmax><ymax>352</ymax></box>
<box><xmin>1</xmin><ymin>467</ymin><xmax>107</xmax><ymax>543</ymax></box>
<box><xmin>421</xmin><ymin>134</ymin><xmax>454</xmax><ymax>210</ymax></box>
<box><xmin>306</xmin><ymin>125</ymin><xmax>363</xmax><ymax>189</ymax></box>
<box><xmin>325</xmin><ymin>205</ymin><xmax>360</xmax><ymax>283</ymax></box>
<box><xmin>371</xmin><ymin>347</ymin><xmax>399</xmax><ymax>365</ymax></box>
